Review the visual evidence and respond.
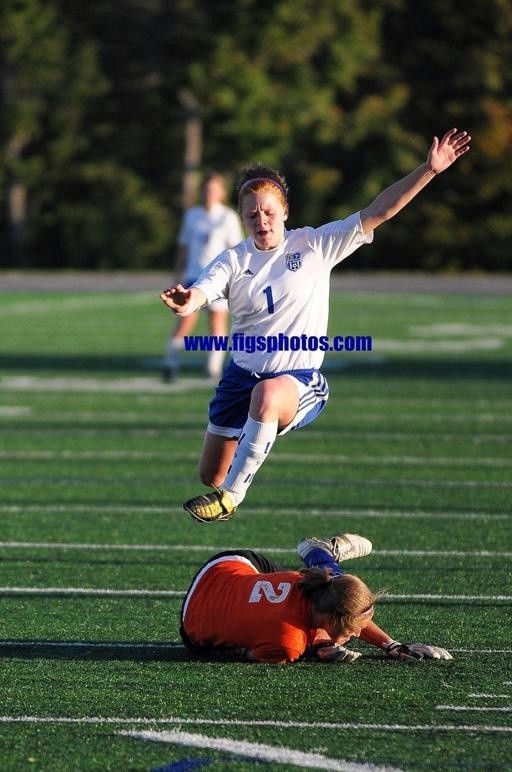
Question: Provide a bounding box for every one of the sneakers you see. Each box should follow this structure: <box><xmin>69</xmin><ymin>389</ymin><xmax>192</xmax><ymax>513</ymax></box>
<box><xmin>183</xmin><ymin>489</ymin><xmax>236</xmax><ymax>523</ymax></box>
<box><xmin>297</xmin><ymin>532</ymin><xmax>374</xmax><ymax>563</ymax></box>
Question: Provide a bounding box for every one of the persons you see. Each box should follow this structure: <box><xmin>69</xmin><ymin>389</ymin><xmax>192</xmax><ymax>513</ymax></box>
<box><xmin>179</xmin><ymin>531</ymin><xmax>454</xmax><ymax>664</ymax></box>
<box><xmin>158</xmin><ymin>128</ymin><xmax>472</xmax><ymax>525</ymax></box>
<box><xmin>160</xmin><ymin>172</ymin><xmax>245</xmax><ymax>386</ymax></box>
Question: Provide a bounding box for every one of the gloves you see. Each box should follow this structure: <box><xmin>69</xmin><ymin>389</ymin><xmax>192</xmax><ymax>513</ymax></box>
<box><xmin>314</xmin><ymin>643</ymin><xmax>362</xmax><ymax>663</ymax></box>
<box><xmin>383</xmin><ymin>639</ymin><xmax>454</xmax><ymax>662</ymax></box>
